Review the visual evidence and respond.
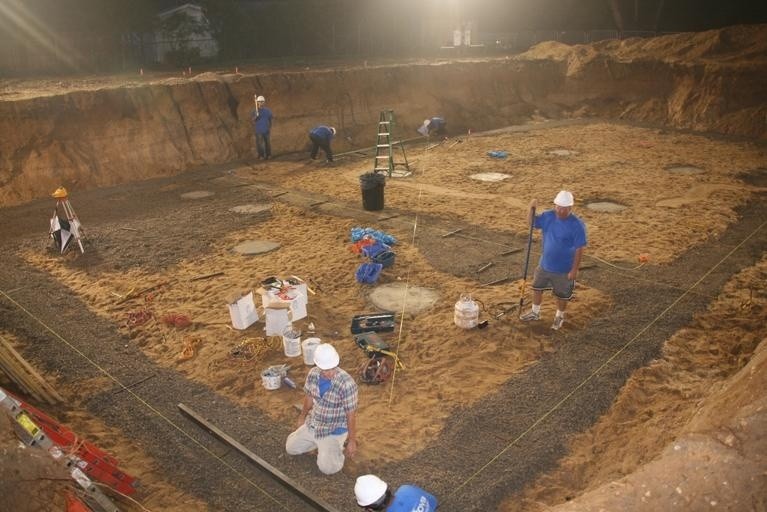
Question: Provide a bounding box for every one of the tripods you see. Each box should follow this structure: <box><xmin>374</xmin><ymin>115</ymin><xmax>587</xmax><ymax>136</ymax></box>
<box><xmin>45</xmin><ymin>197</ymin><xmax>89</xmax><ymax>255</ymax></box>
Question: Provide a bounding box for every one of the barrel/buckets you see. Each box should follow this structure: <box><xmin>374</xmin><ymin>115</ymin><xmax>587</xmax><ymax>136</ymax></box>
<box><xmin>259</xmin><ymin>369</ymin><xmax>281</xmax><ymax>390</ymax></box>
<box><xmin>282</xmin><ymin>330</ymin><xmax>302</xmax><ymax>357</ymax></box>
<box><xmin>384</xmin><ymin>484</ymin><xmax>438</xmax><ymax>512</ymax></box>
<box><xmin>302</xmin><ymin>337</ymin><xmax>322</xmax><ymax>366</ymax></box>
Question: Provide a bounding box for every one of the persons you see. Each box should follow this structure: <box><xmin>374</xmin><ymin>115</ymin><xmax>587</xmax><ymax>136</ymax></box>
<box><xmin>284</xmin><ymin>342</ymin><xmax>358</xmax><ymax>474</ymax></box>
<box><xmin>518</xmin><ymin>190</ymin><xmax>587</xmax><ymax>330</ymax></box>
<box><xmin>252</xmin><ymin>96</ymin><xmax>273</xmax><ymax>161</ymax></box>
<box><xmin>352</xmin><ymin>473</ymin><xmax>439</xmax><ymax>511</ymax></box>
<box><xmin>422</xmin><ymin>117</ymin><xmax>448</xmax><ymax>136</ymax></box>
<box><xmin>307</xmin><ymin>126</ymin><xmax>336</xmax><ymax>162</ymax></box>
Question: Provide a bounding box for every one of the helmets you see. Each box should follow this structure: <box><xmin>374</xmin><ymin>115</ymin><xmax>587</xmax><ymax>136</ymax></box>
<box><xmin>554</xmin><ymin>190</ymin><xmax>574</xmax><ymax>206</ymax></box>
<box><xmin>53</xmin><ymin>188</ymin><xmax>66</xmax><ymax>198</ymax></box>
<box><xmin>313</xmin><ymin>344</ymin><xmax>341</xmax><ymax>372</ymax></box>
<box><xmin>331</xmin><ymin>127</ymin><xmax>336</xmax><ymax>135</ymax></box>
<box><xmin>257</xmin><ymin>96</ymin><xmax>265</xmax><ymax>102</ymax></box>
<box><xmin>355</xmin><ymin>475</ymin><xmax>389</xmax><ymax>508</ymax></box>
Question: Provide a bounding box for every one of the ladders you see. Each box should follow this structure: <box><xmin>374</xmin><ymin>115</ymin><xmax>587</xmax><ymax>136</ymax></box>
<box><xmin>373</xmin><ymin>109</ymin><xmax>411</xmax><ymax>176</ymax></box>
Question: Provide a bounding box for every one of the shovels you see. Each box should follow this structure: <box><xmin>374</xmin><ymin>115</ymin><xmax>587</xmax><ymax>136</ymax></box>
<box><xmin>448</xmin><ymin>139</ymin><xmax>462</xmax><ymax>149</ymax></box>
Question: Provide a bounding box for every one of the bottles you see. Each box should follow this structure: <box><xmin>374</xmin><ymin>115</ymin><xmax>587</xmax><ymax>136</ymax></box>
<box><xmin>282</xmin><ymin>376</ymin><xmax>297</xmax><ymax>390</ymax></box>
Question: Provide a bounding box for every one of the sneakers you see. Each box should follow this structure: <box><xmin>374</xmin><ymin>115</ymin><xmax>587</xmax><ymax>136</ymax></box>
<box><xmin>519</xmin><ymin>310</ymin><xmax>541</xmax><ymax>321</ymax></box>
<box><xmin>551</xmin><ymin>316</ymin><xmax>564</xmax><ymax>331</ymax></box>
<box><xmin>259</xmin><ymin>155</ymin><xmax>272</xmax><ymax>160</ymax></box>
<box><xmin>327</xmin><ymin>161</ymin><xmax>336</xmax><ymax>167</ymax></box>
